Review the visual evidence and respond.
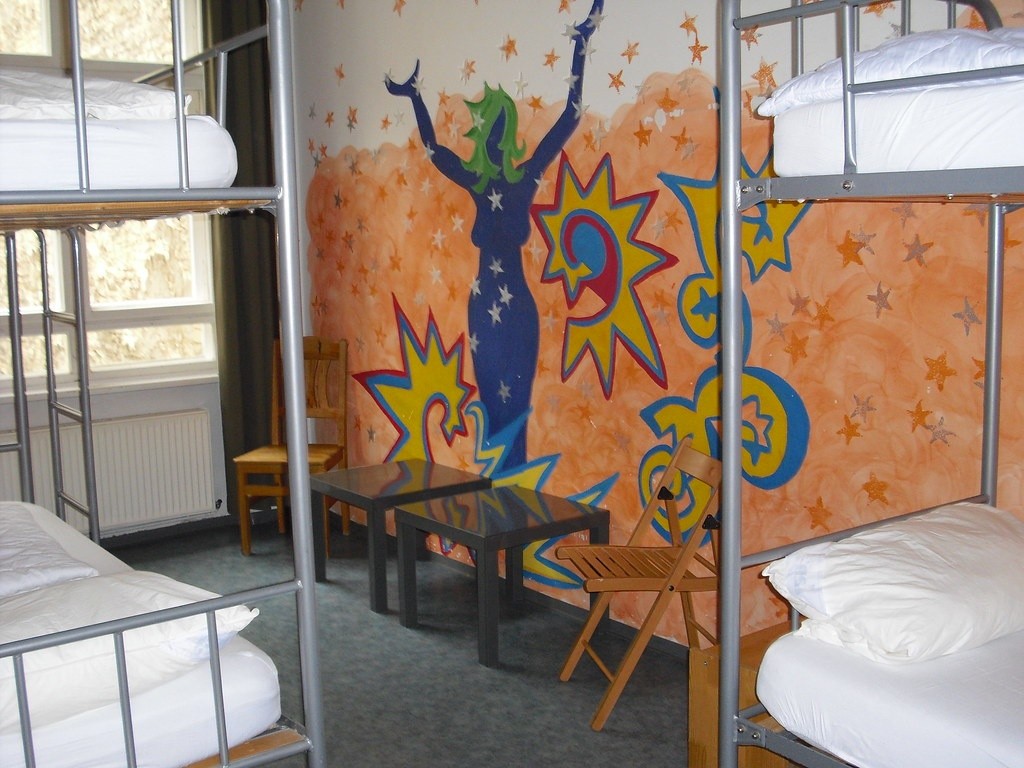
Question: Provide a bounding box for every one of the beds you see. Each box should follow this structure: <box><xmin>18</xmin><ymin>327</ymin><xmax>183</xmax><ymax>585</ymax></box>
<box><xmin>0</xmin><ymin>0</ymin><xmax>327</xmax><ymax>768</ymax></box>
<box><xmin>714</xmin><ymin>0</ymin><xmax>1024</xmax><ymax>768</ymax></box>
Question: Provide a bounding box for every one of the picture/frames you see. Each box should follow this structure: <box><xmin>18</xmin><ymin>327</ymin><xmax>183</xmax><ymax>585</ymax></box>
<box><xmin>0</xmin><ymin>406</ymin><xmax>216</xmax><ymax>531</ymax></box>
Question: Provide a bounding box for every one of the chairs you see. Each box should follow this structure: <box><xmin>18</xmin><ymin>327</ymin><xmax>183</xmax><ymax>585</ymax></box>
<box><xmin>553</xmin><ymin>436</ymin><xmax>723</xmax><ymax>730</ymax></box>
<box><xmin>233</xmin><ymin>334</ymin><xmax>349</xmax><ymax>559</ymax></box>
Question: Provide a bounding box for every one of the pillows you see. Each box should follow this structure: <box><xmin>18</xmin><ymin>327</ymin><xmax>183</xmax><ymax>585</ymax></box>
<box><xmin>760</xmin><ymin>502</ymin><xmax>1024</xmax><ymax>664</ymax></box>
<box><xmin>1</xmin><ymin>570</ymin><xmax>261</xmax><ymax>738</ymax></box>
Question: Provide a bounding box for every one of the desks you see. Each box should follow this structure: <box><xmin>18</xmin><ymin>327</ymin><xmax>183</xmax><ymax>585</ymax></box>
<box><xmin>310</xmin><ymin>457</ymin><xmax>492</xmax><ymax>614</ymax></box>
<box><xmin>392</xmin><ymin>485</ymin><xmax>610</xmax><ymax>668</ymax></box>
